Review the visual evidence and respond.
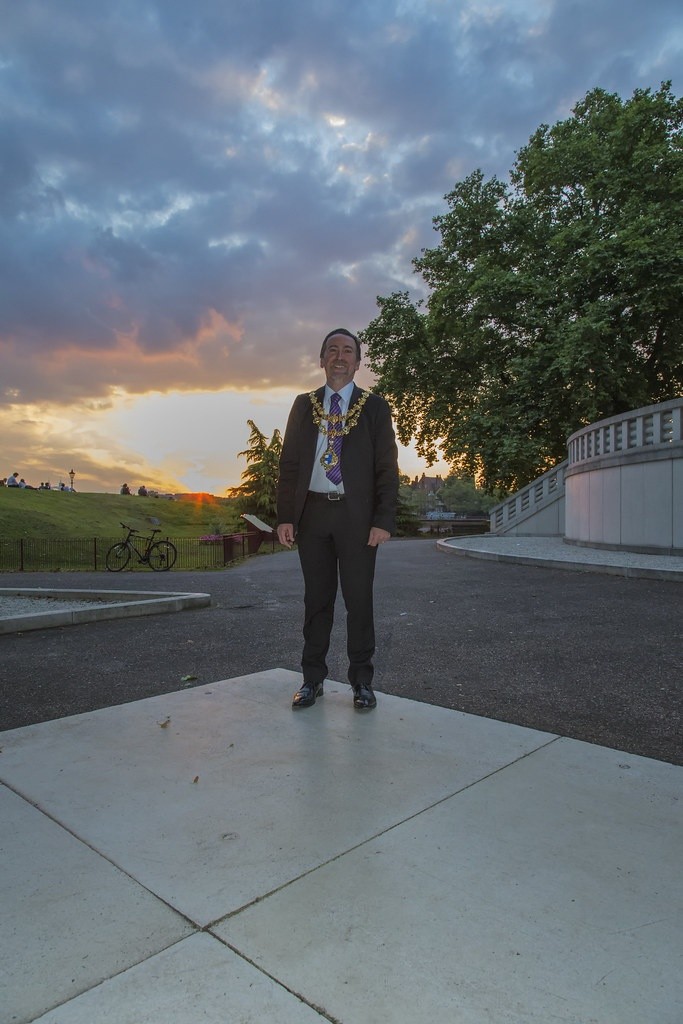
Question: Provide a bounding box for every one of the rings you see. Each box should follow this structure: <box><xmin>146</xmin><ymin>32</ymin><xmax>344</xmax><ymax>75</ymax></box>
<box><xmin>382</xmin><ymin>541</ymin><xmax>385</xmax><ymax>544</ymax></box>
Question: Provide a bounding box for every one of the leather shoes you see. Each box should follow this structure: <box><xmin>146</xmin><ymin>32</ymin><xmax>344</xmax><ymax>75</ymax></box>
<box><xmin>352</xmin><ymin>682</ymin><xmax>377</xmax><ymax>712</ymax></box>
<box><xmin>291</xmin><ymin>681</ymin><xmax>324</xmax><ymax>708</ymax></box>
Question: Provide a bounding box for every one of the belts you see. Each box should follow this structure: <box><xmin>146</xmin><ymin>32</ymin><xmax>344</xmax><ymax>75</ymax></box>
<box><xmin>307</xmin><ymin>490</ymin><xmax>345</xmax><ymax>506</ymax></box>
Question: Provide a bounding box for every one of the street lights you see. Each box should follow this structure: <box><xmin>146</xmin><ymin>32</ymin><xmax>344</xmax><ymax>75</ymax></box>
<box><xmin>68</xmin><ymin>469</ymin><xmax>75</xmax><ymax>491</ymax></box>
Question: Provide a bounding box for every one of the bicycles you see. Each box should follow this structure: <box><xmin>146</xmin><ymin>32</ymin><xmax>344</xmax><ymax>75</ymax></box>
<box><xmin>106</xmin><ymin>522</ymin><xmax>178</xmax><ymax>572</ymax></box>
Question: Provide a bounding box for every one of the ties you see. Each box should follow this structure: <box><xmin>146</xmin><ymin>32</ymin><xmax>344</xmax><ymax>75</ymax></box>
<box><xmin>326</xmin><ymin>393</ymin><xmax>342</xmax><ymax>486</ymax></box>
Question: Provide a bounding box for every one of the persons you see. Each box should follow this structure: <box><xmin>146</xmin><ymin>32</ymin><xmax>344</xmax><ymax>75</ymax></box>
<box><xmin>0</xmin><ymin>473</ymin><xmax>75</xmax><ymax>491</ymax></box>
<box><xmin>121</xmin><ymin>483</ymin><xmax>175</xmax><ymax>500</ymax></box>
<box><xmin>274</xmin><ymin>328</ymin><xmax>400</xmax><ymax>712</ymax></box>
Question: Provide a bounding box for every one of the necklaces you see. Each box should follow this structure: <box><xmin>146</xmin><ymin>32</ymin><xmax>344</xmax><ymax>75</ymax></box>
<box><xmin>309</xmin><ymin>391</ymin><xmax>371</xmax><ymax>473</ymax></box>
